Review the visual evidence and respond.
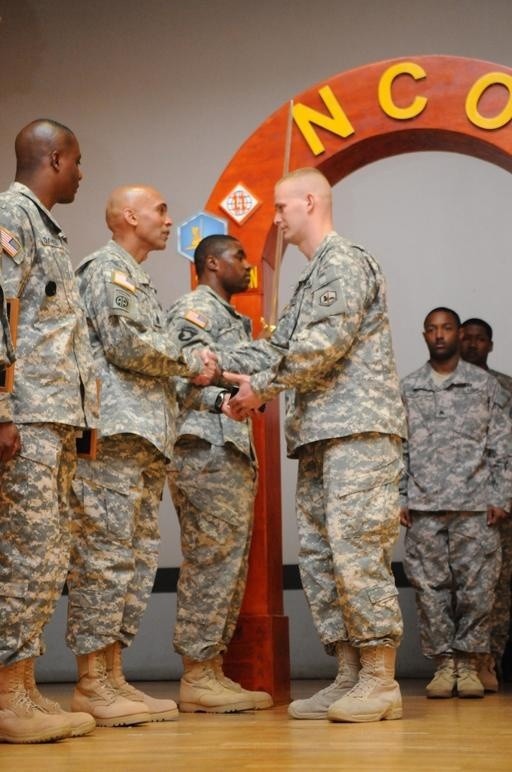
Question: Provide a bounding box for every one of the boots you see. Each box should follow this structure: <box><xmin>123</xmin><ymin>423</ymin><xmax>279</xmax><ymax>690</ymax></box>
<box><xmin>22</xmin><ymin>659</ymin><xmax>94</xmax><ymax>737</ymax></box>
<box><xmin>330</xmin><ymin>638</ymin><xmax>403</xmax><ymax>720</ymax></box>
<box><xmin>424</xmin><ymin>652</ymin><xmax>455</xmax><ymax>698</ymax></box>
<box><xmin>70</xmin><ymin>652</ymin><xmax>149</xmax><ymax>725</ymax></box>
<box><xmin>178</xmin><ymin>650</ymin><xmax>252</xmax><ymax>714</ymax></box>
<box><xmin>103</xmin><ymin>642</ymin><xmax>178</xmax><ymax>719</ymax></box>
<box><xmin>456</xmin><ymin>656</ymin><xmax>482</xmax><ymax>695</ymax></box>
<box><xmin>288</xmin><ymin>642</ymin><xmax>358</xmax><ymax>719</ymax></box>
<box><xmin>205</xmin><ymin>651</ymin><xmax>276</xmax><ymax>709</ymax></box>
<box><xmin>483</xmin><ymin>658</ymin><xmax>502</xmax><ymax>692</ymax></box>
<box><xmin>0</xmin><ymin>665</ymin><xmax>71</xmax><ymax>744</ymax></box>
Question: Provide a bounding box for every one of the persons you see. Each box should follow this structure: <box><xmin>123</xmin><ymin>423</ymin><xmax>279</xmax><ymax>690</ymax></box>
<box><xmin>460</xmin><ymin>316</ymin><xmax>512</xmax><ymax>692</ymax></box>
<box><xmin>192</xmin><ymin>165</ymin><xmax>406</xmax><ymax>724</ymax></box>
<box><xmin>163</xmin><ymin>235</ymin><xmax>273</xmax><ymax>713</ymax></box>
<box><xmin>0</xmin><ymin>118</ymin><xmax>103</xmax><ymax>745</ymax></box>
<box><xmin>397</xmin><ymin>307</ymin><xmax>512</xmax><ymax>698</ymax></box>
<box><xmin>67</xmin><ymin>183</ymin><xmax>218</xmax><ymax>728</ymax></box>
